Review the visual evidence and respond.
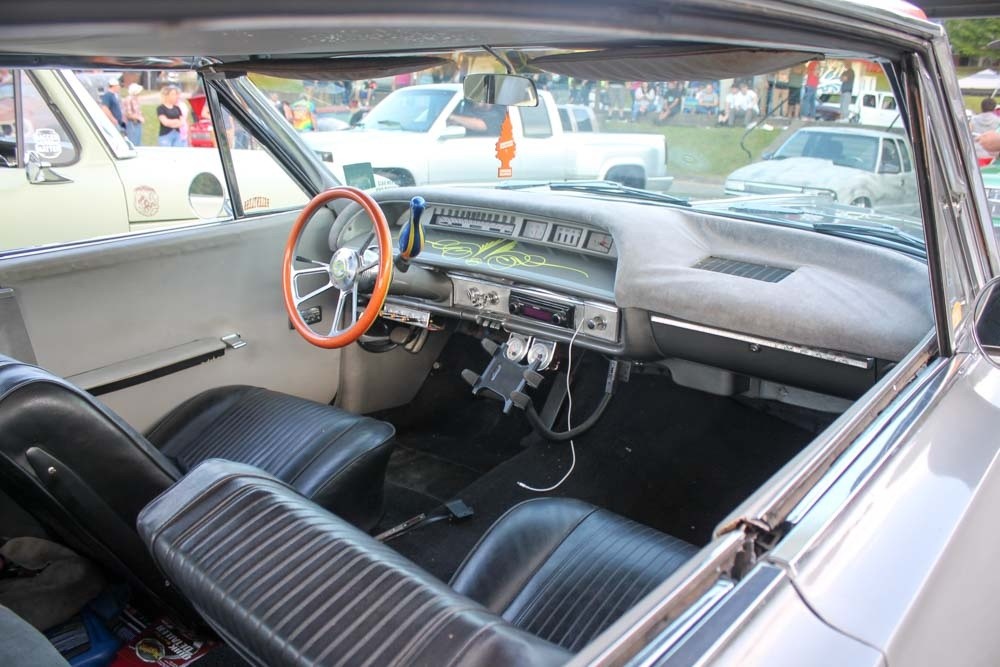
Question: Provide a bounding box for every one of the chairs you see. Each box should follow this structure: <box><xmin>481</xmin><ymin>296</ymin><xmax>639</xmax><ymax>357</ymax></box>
<box><xmin>137</xmin><ymin>457</ymin><xmax>707</xmax><ymax>667</ymax></box>
<box><xmin>822</xmin><ymin>142</ymin><xmax>843</xmax><ymax>157</ymax></box>
<box><xmin>0</xmin><ymin>352</ymin><xmax>396</xmax><ymax>594</ymax></box>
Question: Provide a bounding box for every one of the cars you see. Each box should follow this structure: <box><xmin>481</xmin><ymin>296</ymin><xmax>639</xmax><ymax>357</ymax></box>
<box><xmin>0</xmin><ymin>66</ymin><xmax>401</xmax><ymax>255</ymax></box>
<box><xmin>811</xmin><ymin>88</ymin><xmax>907</xmax><ymax>129</ymax></box>
<box><xmin>0</xmin><ymin>0</ymin><xmax>1000</xmax><ymax>667</ymax></box>
<box><xmin>724</xmin><ymin>124</ymin><xmax>918</xmax><ymax>209</ymax></box>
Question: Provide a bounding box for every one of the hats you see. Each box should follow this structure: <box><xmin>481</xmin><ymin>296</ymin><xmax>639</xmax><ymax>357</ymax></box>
<box><xmin>129</xmin><ymin>83</ymin><xmax>143</xmax><ymax>94</ymax></box>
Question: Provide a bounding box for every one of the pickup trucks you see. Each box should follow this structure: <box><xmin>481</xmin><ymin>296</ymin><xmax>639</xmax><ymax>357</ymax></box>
<box><xmin>305</xmin><ymin>81</ymin><xmax>674</xmax><ymax>192</ymax></box>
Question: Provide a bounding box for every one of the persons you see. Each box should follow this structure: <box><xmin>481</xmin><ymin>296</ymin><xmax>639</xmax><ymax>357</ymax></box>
<box><xmin>269</xmin><ymin>80</ymin><xmax>375</xmax><ymax>131</ymax></box>
<box><xmin>787</xmin><ymin>60</ymin><xmax>820</xmax><ymax>120</ymax></box>
<box><xmin>447</xmin><ymin>105</ymin><xmax>504</xmax><ymax>137</ymax></box>
<box><xmin>697</xmin><ymin>84</ymin><xmax>718</xmax><ymax>119</ymax></box>
<box><xmin>970</xmin><ymin>98</ymin><xmax>1000</xmax><ymax>168</ymax></box>
<box><xmin>392</xmin><ymin>61</ymin><xmax>459</xmax><ymax>91</ymax></box>
<box><xmin>629</xmin><ymin>82</ymin><xmax>685</xmax><ymax>125</ymax></box>
<box><xmin>101</xmin><ymin>81</ymin><xmax>144</xmax><ymax>146</ymax></box>
<box><xmin>720</xmin><ymin>83</ymin><xmax>758</xmax><ymax>128</ymax></box>
<box><xmin>533</xmin><ymin>74</ymin><xmax>627</xmax><ymax>121</ymax></box>
<box><xmin>201</xmin><ymin>102</ymin><xmax>235</xmax><ymax>147</ymax></box>
<box><xmin>840</xmin><ymin>62</ymin><xmax>855</xmax><ymax>120</ymax></box>
<box><xmin>156</xmin><ymin>88</ymin><xmax>190</xmax><ymax>147</ymax></box>
